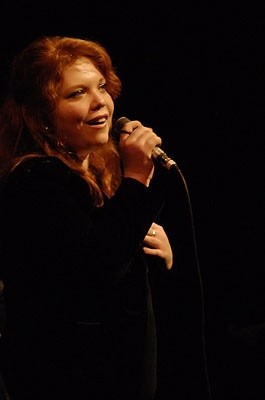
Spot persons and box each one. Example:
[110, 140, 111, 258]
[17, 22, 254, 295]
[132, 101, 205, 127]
[0, 35, 174, 400]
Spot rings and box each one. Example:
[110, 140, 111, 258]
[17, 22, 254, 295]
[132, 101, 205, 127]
[151, 229, 156, 237]
[120, 130, 133, 135]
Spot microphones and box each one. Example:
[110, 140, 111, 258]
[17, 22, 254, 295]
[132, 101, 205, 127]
[115, 117, 176, 171]
